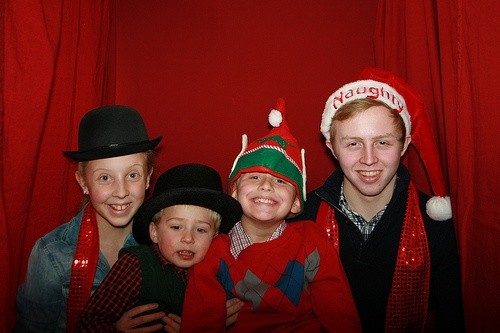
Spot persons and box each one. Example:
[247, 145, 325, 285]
[74, 163, 243, 333]
[15, 106, 182, 333]
[180, 109, 362, 333]
[226, 69, 466, 333]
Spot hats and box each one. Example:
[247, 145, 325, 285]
[132, 164, 243, 248]
[63, 105, 162, 162]
[227, 98, 306, 218]
[319, 66, 453, 221]
